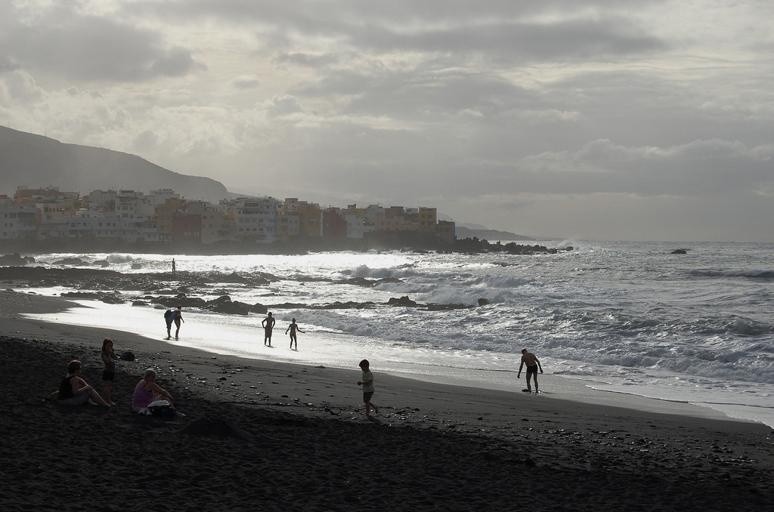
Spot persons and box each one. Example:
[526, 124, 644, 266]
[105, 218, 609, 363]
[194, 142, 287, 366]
[131, 369, 186, 417]
[172, 258, 175, 272]
[262, 312, 275, 347]
[57, 360, 109, 407]
[164, 310, 173, 339]
[99, 338, 119, 406]
[517, 349, 543, 394]
[285, 318, 305, 351]
[357, 359, 378, 415]
[173, 306, 184, 341]
